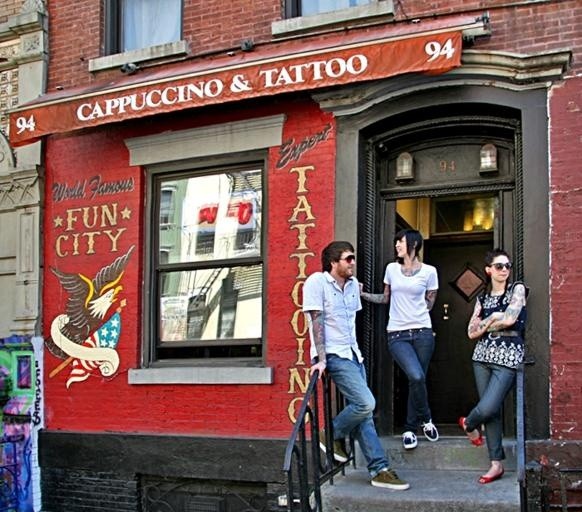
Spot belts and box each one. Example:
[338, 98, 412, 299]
[486, 329, 520, 338]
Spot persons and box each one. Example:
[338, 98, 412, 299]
[459, 248, 529, 483]
[303, 241, 410, 491]
[359, 229, 439, 450]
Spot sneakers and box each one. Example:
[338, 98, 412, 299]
[402, 431, 419, 451]
[370, 467, 411, 491]
[319, 429, 349, 462]
[420, 419, 441, 442]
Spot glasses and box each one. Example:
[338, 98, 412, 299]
[489, 261, 513, 271]
[338, 255, 356, 265]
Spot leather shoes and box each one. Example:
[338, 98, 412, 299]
[457, 415, 485, 446]
[477, 469, 505, 484]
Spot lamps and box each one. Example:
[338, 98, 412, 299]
[478, 143, 499, 176]
[394, 152, 416, 183]
